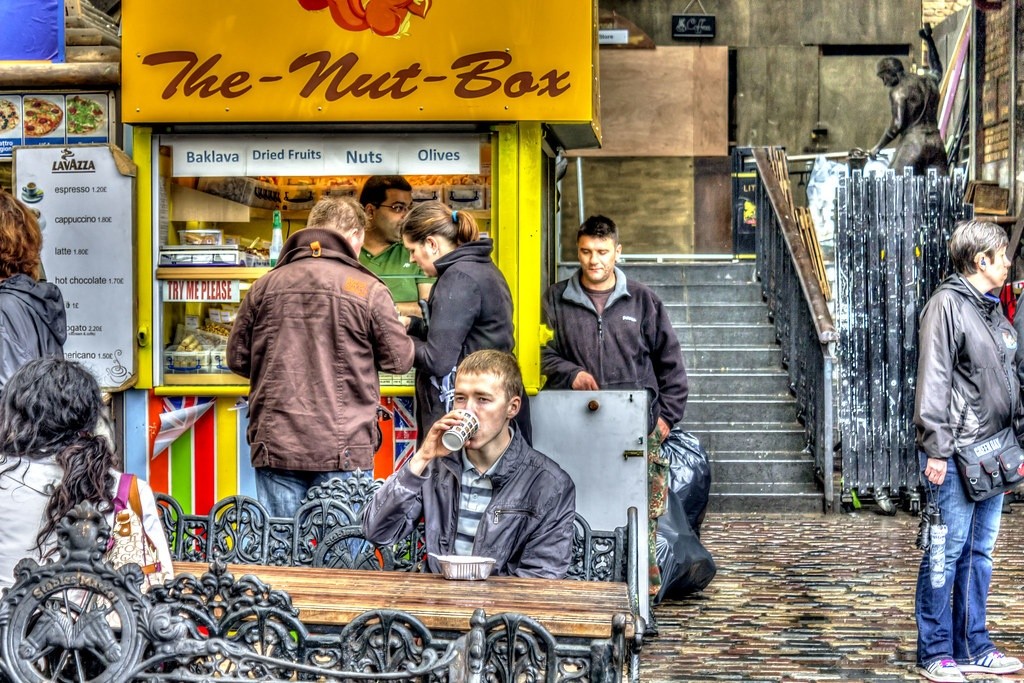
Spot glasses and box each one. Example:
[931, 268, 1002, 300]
[378, 204, 414, 213]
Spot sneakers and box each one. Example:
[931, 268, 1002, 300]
[915, 657, 964, 683]
[956, 650, 1023, 674]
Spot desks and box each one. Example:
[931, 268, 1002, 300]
[148, 562, 634, 683]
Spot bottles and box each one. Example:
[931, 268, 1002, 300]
[269, 210, 283, 268]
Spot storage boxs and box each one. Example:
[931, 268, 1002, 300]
[197, 175, 491, 210]
[164, 343, 227, 369]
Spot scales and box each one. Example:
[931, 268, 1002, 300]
[159, 244, 246, 267]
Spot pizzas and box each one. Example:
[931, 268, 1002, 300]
[0, 99, 19, 133]
[23, 97, 62, 136]
[66, 96, 105, 135]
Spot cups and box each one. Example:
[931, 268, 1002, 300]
[28, 207, 41, 221]
[443, 408, 480, 452]
[21, 182, 38, 197]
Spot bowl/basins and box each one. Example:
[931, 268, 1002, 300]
[437, 555, 497, 581]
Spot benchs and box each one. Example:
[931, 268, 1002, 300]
[0, 467, 645, 683]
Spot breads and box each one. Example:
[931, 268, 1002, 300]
[211, 175, 481, 188]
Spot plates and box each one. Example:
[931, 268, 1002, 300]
[36, 215, 47, 229]
[21, 189, 44, 202]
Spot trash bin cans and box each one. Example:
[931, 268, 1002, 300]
[731, 146, 787, 260]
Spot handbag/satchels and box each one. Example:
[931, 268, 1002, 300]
[953, 427, 1024, 502]
[97, 473, 165, 631]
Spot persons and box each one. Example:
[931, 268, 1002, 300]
[0, 361, 176, 683]
[913, 220, 1024, 683]
[0, 187, 67, 397]
[226, 175, 532, 569]
[868, 22, 947, 176]
[540, 215, 689, 637]
[361, 350, 576, 580]
